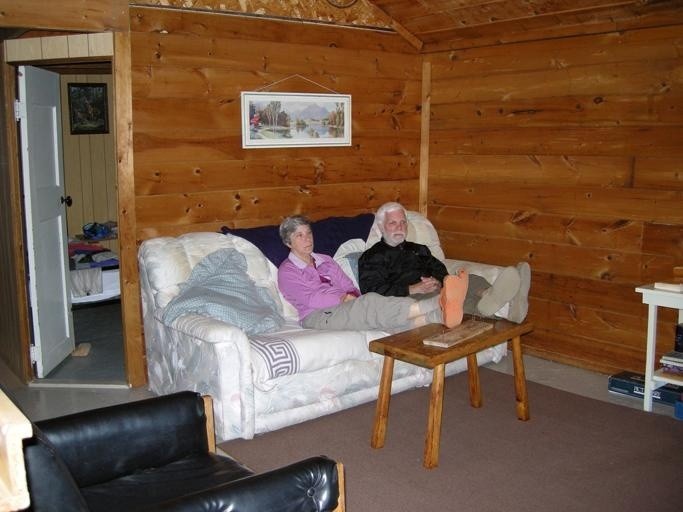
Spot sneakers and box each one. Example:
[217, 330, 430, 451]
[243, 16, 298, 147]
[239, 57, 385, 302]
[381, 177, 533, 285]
[476, 262, 531, 324]
[438, 267, 469, 329]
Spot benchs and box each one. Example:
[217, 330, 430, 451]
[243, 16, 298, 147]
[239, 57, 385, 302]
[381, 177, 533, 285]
[369, 313, 536, 468]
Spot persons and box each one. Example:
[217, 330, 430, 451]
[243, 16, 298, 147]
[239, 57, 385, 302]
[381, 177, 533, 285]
[355, 200, 531, 325]
[273, 213, 470, 335]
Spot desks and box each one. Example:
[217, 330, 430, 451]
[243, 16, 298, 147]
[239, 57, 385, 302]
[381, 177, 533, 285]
[635, 283, 683, 412]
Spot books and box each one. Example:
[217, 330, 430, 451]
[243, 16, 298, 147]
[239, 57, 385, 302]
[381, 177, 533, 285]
[652, 276, 682, 293]
[657, 349, 682, 377]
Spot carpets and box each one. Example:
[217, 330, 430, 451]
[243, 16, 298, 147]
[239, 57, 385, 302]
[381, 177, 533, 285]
[217, 363, 683, 512]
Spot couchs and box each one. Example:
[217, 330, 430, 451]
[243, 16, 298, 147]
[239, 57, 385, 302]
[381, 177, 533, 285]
[138, 209, 511, 442]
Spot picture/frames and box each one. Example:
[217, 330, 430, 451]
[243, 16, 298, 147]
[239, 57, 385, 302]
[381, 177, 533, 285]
[241, 91, 352, 150]
[67, 82, 109, 135]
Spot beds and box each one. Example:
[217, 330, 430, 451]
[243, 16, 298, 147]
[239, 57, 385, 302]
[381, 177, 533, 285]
[68, 241, 121, 303]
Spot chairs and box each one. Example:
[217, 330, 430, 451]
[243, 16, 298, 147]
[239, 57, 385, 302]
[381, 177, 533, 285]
[1, 388, 346, 512]
